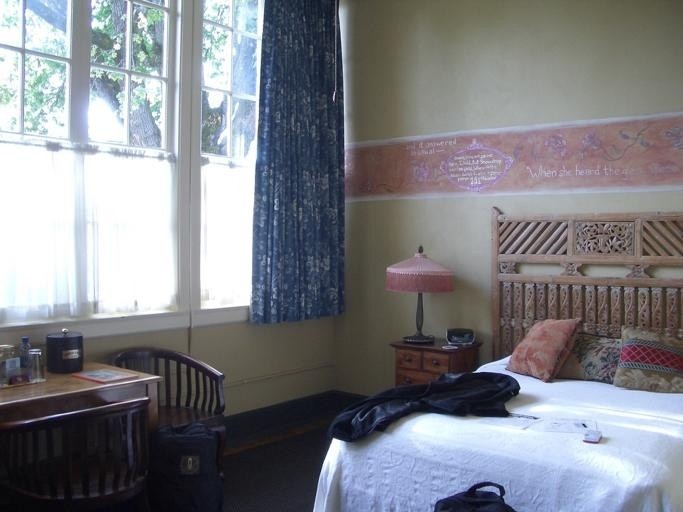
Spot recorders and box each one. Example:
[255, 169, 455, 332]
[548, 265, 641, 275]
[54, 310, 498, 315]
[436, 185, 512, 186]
[446, 328, 475, 345]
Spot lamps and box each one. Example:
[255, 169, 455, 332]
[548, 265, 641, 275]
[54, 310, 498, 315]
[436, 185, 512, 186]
[383, 244, 454, 346]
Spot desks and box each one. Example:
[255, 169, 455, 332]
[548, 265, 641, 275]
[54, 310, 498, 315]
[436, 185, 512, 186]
[1, 361, 163, 511]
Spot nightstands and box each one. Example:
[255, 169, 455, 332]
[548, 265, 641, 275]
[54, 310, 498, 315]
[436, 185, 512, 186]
[389, 338, 482, 386]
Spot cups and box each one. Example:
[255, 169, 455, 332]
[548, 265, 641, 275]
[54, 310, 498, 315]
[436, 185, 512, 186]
[26, 348, 46, 383]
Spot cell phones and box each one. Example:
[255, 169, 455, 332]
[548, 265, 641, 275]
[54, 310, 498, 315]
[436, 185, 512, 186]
[583, 429, 602, 442]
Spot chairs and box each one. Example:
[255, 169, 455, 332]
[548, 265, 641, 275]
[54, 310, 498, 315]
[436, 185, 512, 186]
[0, 395, 151, 510]
[110, 344, 230, 484]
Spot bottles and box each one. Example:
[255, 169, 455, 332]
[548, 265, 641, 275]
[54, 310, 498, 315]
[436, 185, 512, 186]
[18, 336, 32, 384]
[0, 344, 18, 388]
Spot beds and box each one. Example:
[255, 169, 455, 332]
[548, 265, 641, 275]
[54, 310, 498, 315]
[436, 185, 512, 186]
[312, 202, 682, 511]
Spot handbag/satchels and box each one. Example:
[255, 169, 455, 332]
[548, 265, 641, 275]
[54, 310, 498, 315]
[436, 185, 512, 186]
[433, 479, 517, 512]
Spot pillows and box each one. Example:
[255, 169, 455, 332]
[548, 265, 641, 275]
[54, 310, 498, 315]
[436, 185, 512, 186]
[611, 324, 683, 392]
[502, 316, 583, 383]
[555, 331, 622, 384]
